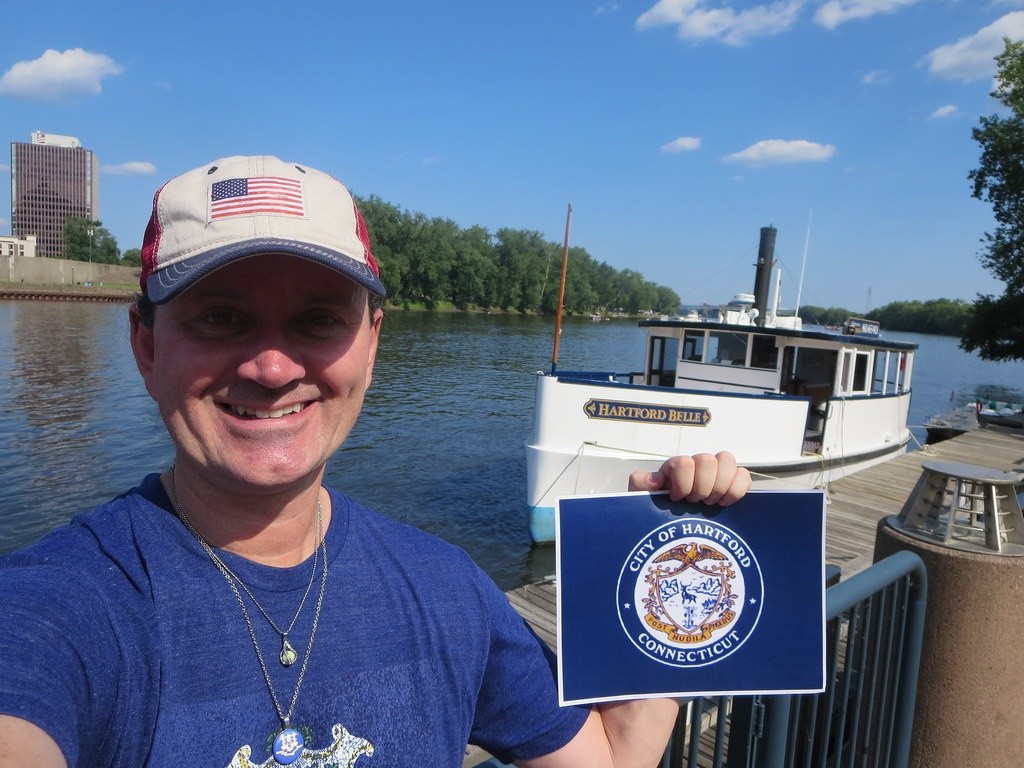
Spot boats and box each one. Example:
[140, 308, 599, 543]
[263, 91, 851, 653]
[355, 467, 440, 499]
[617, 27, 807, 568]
[717, 294, 803, 330]
[589, 313, 602, 320]
[522, 226, 922, 545]
[975, 407, 1024, 429]
[647, 310, 711, 322]
[842, 316, 880, 339]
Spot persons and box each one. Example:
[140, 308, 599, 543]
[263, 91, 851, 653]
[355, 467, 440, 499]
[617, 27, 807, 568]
[0, 155, 752, 768]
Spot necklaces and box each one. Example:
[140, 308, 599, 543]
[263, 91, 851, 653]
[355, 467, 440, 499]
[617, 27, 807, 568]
[170, 464, 328, 765]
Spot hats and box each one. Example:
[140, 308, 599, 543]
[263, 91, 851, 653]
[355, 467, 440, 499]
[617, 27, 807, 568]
[139, 155, 388, 307]
